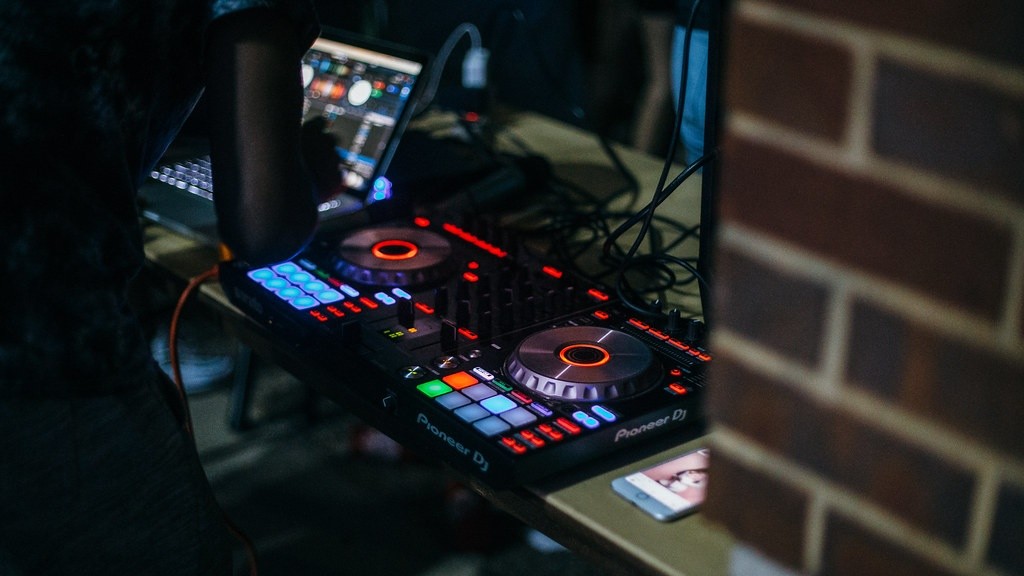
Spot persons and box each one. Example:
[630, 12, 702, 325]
[0, 3, 351, 576]
[657, 467, 709, 496]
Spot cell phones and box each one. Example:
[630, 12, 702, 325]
[609, 445, 708, 524]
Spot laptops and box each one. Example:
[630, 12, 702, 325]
[133, 24, 437, 251]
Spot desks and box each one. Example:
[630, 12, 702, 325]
[141, 104, 731, 576]
